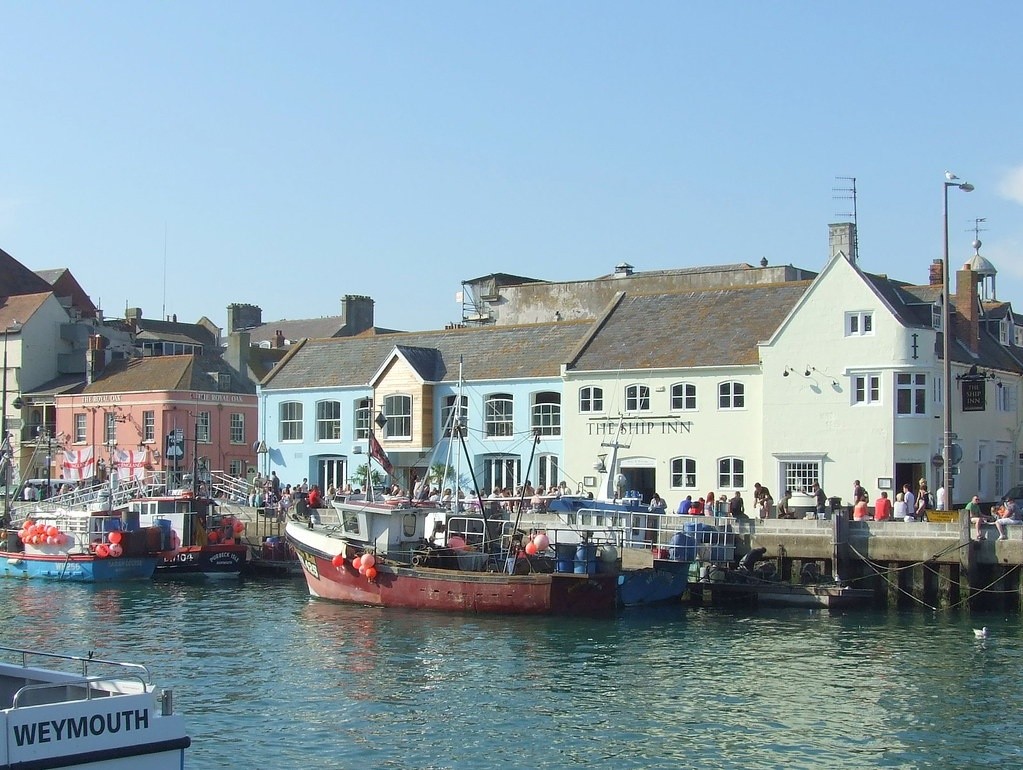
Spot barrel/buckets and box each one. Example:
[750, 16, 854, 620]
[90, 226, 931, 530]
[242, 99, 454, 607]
[102, 518, 120, 548]
[121, 511, 139, 532]
[265, 537, 279, 543]
[806, 512, 814, 520]
[817, 513, 825, 520]
[573, 543, 597, 574]
[153, 519, 171, 551]
[555, 543, 578, 573]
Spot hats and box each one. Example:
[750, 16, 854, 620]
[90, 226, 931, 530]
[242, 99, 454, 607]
[1005, 497, 1014, 502]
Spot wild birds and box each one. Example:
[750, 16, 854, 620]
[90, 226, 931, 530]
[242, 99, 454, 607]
[972, 626, 988, 637]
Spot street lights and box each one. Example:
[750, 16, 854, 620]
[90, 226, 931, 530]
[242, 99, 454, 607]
[944, 181, 974, 511]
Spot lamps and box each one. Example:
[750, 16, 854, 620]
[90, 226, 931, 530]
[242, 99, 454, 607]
[805, 365, 839, 388]
[784, 366, 818, 389]
[987, 377, 1003, 389]
[984, 368, 995, 380]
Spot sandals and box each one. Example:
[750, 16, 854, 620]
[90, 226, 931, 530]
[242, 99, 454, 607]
[977, 535, 986, 540]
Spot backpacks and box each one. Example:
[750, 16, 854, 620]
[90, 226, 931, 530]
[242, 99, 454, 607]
[691, 501, 700, 508]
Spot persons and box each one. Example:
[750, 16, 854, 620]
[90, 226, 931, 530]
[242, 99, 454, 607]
[802, 482, 828, 520]
[12, 474, 109, 503]
[853, 480, 871, 522]
[224, 523, 234, 545]
[777, 490, 797, 520]
[676, 492, 750, 520]
[384, 475, 595, 513]
[754, 482, 774, 519]
[199, 479, 210, 496]
[874, 478, 945, 523]
[647, 491, 668, 549]
[965, 495, 1022, 540]
[220, 471, 366, 520]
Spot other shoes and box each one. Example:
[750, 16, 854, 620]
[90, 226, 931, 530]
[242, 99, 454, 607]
[998, 534, 1006, 539]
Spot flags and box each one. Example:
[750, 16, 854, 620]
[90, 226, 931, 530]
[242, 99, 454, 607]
[64, 444, 94, 480]
[112, 449, 147, 482]
[370, 432, 393, 475]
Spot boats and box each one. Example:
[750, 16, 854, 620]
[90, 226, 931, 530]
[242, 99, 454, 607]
[0, 522, 163, 585]
[283, 515, 622, 618]
[0, 641, 193, 770]
[686, 556, 875, 611]
[0, 385, 694, 609]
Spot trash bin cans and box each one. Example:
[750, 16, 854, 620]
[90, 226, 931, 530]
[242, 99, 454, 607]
[7, 531, 19, 552]
[574, 543, 598, 574]
[153, 519, 171, 551]
[146, 527, 161, 553]
[555, 542, 577, 573]
[125, 532, 147, 555]
